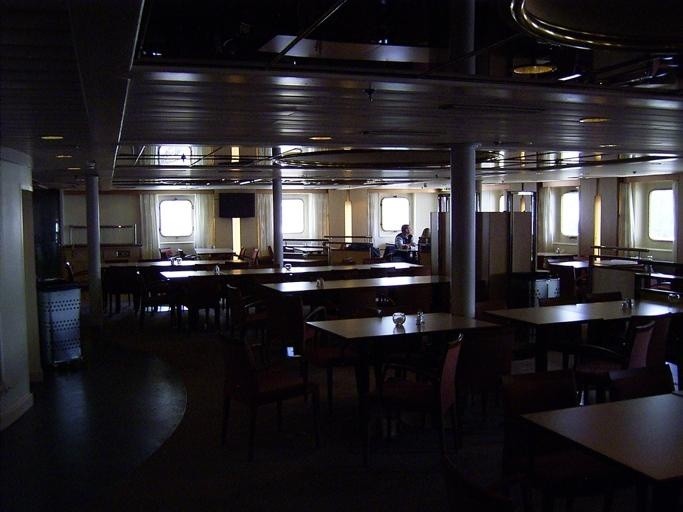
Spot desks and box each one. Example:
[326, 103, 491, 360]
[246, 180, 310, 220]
[524, 388, 683, 512]
[393, 245, 420, 264]
[193, 247, 238, 260]
[304, 311, 499, 428]
[537, 248, 578, 268]
[98, 258, 249, 269]
[633, 268, 683, 301]
[483, 299, 683, 373]
[295, 247, 324, 256]
[265, 273, 453, 298]
[158, 262, 424, 281]
[551, 258, 646, 298]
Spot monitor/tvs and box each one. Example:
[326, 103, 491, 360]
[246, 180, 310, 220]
[219, 192, 255, 218]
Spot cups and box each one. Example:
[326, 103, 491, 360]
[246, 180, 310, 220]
[393, 313, 406, 325]
[285, 264, 291, 270]
[177, 258, 182, 264]
[393, 325, 405, 334]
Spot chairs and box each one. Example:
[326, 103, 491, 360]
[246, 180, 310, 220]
[244, 248, 259, 268]
[416, 242, 431, 266]
[472, 301, 535, 359]
[107, 267, 137, 314]
[60, 262, 90, 288]
[588, 323, 656, 407]
[611, 364, 675, 401]
[221, 283, 271, 343]
[329, 269, 360, 283]
[224, 332, 326, 468]
[385, 242, 401, 262]
[233, 246, 248, 260]
[502, 367, 618, 512]
[159, 246, 177, 260]
[183, 275, 220, 328]
[624, 314, 671, 363]
[557, 263, 588, 302]
[134, 269, 183, 325]
[264, 294, 328, 344]
[177, 247, 199, 261]
[358, 329, 463, 466]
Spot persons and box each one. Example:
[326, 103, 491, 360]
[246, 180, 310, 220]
[418, 228, 431, 248]
[395, 224, 416, 249]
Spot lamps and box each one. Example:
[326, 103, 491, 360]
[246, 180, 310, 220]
[511, 50, 556, 77]
